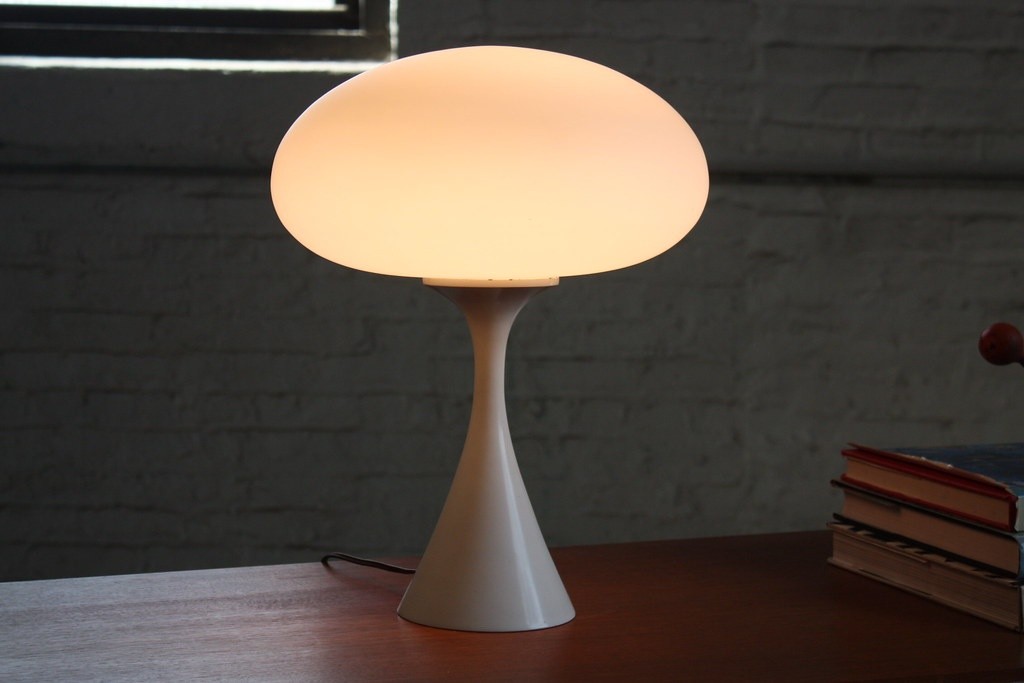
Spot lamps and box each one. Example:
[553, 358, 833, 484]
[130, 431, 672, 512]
[268, 44, 710, 632]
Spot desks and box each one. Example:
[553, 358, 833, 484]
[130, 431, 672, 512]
[1, 529, 1024, 683]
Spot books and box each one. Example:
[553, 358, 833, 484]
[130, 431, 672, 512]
[825, 440, 1024, 628]
